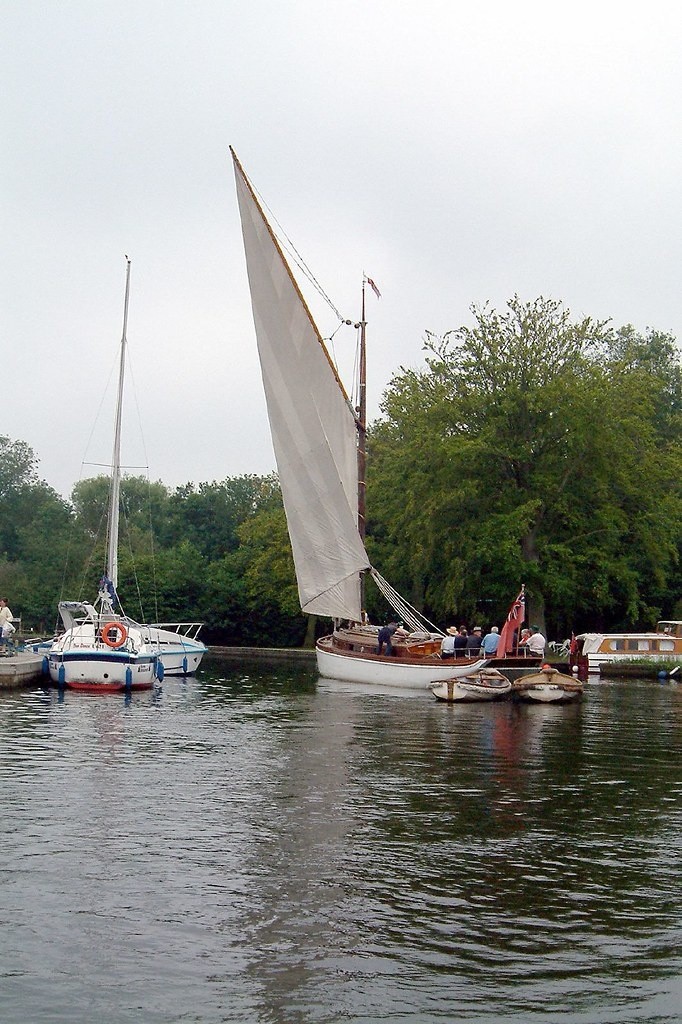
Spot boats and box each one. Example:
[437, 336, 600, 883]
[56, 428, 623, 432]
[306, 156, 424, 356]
[514, 665, 584, 704]
[570, 620, 682, 675]
[427, 669, 514, 703]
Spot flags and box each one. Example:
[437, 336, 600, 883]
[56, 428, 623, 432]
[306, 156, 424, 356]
[495, 590, 525, 660]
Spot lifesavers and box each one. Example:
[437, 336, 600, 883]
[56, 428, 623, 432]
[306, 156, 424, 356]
[102, 623, 126, 647]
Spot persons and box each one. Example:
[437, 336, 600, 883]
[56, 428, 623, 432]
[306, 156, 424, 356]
[441, 625, 545, 660]
[0, 597, 13, 657]
[377, 623, 406, 656]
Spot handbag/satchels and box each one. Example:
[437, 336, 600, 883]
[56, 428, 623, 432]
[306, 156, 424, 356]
[2, 621, 16, 638]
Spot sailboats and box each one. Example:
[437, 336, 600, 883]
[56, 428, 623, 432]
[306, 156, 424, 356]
[228, 143, 543, 692]
[23, 253, 208, 693]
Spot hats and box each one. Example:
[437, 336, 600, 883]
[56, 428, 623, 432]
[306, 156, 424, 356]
[446, 627, 458, 635]
[471, 627, 484, 634]
[529, 625, 540, 632]
